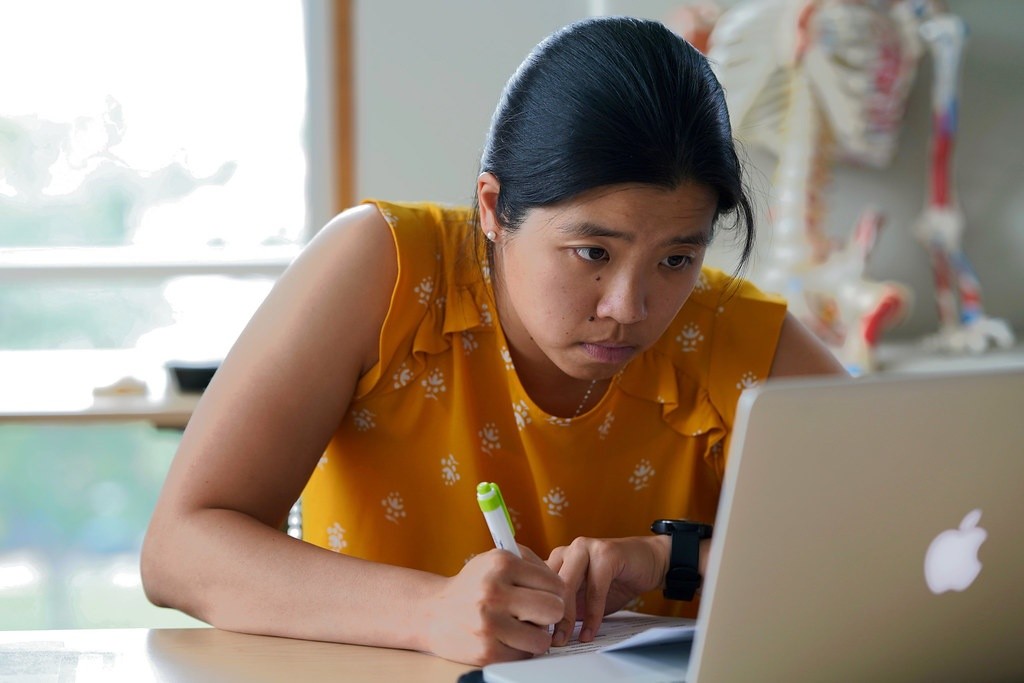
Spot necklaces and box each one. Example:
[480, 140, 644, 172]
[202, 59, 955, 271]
[573, 381, 597, 419]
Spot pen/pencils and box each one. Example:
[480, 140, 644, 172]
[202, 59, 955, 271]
[473, 480, 553, 656]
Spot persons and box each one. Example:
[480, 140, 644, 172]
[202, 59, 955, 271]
[140, 15, 855, 667]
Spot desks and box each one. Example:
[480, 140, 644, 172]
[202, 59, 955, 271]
[0, 590, 483, 683]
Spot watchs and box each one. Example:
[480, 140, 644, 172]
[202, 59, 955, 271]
[650, 519, 713, 602]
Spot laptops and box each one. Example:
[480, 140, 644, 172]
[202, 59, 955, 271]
[483, 356, 1024, 683]
